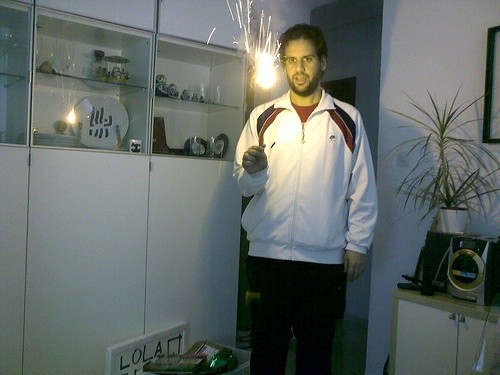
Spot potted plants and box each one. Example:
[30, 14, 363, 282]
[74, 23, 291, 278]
[376, 82, 500, 234]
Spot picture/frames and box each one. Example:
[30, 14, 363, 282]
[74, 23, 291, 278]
[481, 26, 500, 143]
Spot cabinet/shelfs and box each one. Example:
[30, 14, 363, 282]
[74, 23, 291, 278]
[0, 1, 250, 375]
[388, 289, 500, 375]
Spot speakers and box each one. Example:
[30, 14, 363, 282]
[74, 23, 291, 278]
[447, 236, 500, 306]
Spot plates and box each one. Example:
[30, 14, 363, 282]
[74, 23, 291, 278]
[71, 95, 129, 149]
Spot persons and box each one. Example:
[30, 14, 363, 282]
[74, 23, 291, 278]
[232, 24, 379, 375]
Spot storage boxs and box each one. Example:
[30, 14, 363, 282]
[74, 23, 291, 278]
[139, 342, 252, 375]
